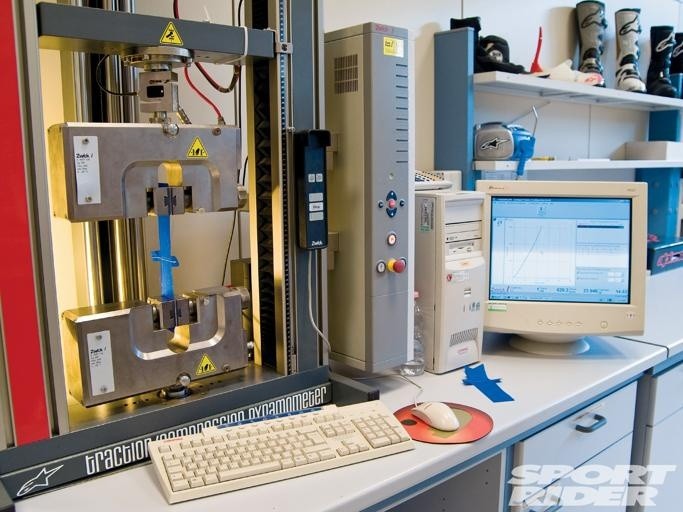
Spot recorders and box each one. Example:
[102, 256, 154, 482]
[473, 100, 551, 162]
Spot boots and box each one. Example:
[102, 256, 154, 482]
[449, 16, 525, 74]
[575, 0, 607, 88]
[614, 7, 646, 93]
[647, 26, 683, 99]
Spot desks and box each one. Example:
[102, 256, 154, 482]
[0, 270, 683, 512]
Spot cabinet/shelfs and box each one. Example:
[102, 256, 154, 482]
[435, 1, 683, 270]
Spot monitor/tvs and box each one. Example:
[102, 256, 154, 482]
[475, 179, 648, 358]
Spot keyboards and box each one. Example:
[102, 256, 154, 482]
[147, 398, 416, 504]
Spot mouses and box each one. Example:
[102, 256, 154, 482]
[411, 401, 460, 431]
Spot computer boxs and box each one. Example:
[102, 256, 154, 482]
[413, 188, 488, 375]
